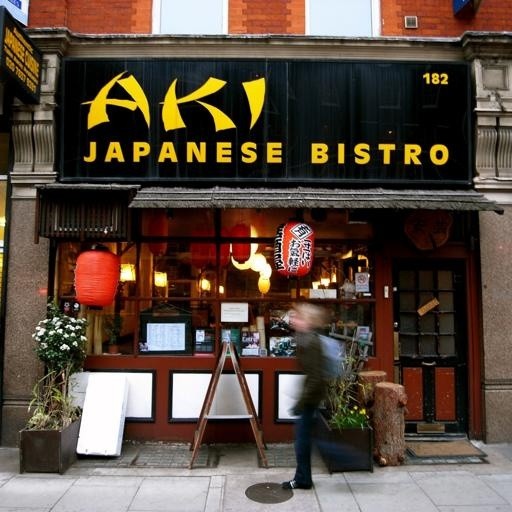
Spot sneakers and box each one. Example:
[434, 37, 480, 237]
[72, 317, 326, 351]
[282, 480, 312, 490]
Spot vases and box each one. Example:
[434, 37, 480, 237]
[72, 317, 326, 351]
[316, 406, 374, 475]
[18, 409, 81, 475]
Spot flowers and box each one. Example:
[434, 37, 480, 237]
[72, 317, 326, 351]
[28, 297, 89, 428]
[327, 328, 373, 432]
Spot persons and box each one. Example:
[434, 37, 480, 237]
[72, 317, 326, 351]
[282, 302, 341, 488]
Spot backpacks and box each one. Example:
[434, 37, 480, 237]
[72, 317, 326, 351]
[317, 333, 342, 379]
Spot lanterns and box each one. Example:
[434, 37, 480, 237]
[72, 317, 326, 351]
[74, 245, 121, 310]
[274, 216, 315, 281]
[191, 223, 250, 269]
[147, 212, 170, 257]
